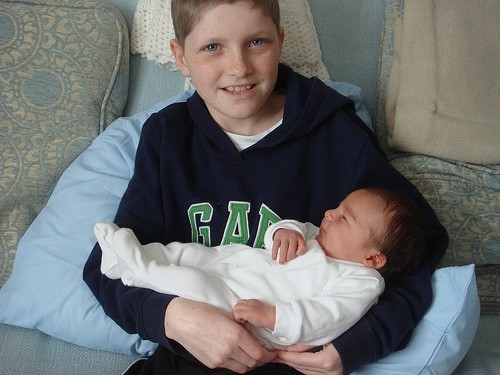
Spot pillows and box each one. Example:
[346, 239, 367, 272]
[0, 82, 480, 375]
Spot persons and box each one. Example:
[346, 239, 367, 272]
[93, 188, 430, 351]
[82, 0, 449, 375]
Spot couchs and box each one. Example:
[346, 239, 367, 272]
[0, 0, 500, 375]
